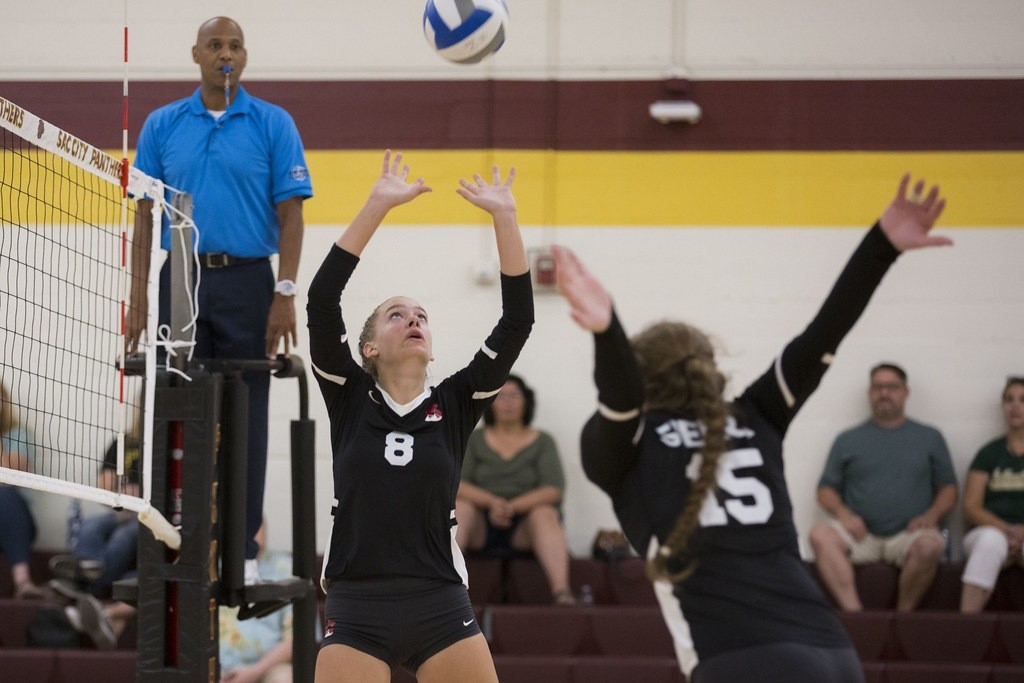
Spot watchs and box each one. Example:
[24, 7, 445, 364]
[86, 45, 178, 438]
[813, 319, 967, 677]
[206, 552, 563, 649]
[274, 279, 298, 297]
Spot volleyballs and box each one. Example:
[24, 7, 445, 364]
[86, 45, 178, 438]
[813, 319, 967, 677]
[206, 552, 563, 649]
[421, 0, 511, 65]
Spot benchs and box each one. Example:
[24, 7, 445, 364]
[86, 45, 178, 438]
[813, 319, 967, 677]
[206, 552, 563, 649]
[0, 554, 1024, 682]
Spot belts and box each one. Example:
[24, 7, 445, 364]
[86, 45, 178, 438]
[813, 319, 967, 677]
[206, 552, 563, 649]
[193, 253, 253, 269]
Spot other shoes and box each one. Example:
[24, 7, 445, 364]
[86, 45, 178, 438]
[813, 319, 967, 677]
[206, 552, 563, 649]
[9, 543, 134, 652]
[551, 589, 577, 609]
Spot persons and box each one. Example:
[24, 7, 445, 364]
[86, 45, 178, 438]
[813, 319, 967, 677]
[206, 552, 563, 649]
[809, 363, 960, 612]
[455, 374, 575, 605]
[961, 376, 1024, 611]
[304, 149, 535, 683]
[552, 172, 956, 683]
[0, 383, 43, 599]
[123, 15, 313, 582]
[50, 423, 266, 651]
